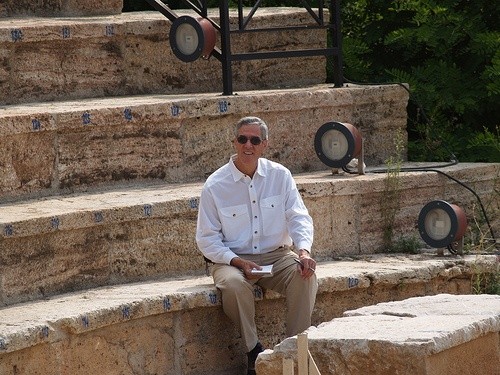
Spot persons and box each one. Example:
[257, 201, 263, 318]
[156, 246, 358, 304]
[196, 116, 320, 374]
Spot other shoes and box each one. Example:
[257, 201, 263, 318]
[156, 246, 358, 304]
[247, 343, 263, 375]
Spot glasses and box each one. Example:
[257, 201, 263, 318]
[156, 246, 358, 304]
[235, 134, 266, 146]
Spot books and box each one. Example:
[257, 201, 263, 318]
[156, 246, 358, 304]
[250, 265, 274, 278]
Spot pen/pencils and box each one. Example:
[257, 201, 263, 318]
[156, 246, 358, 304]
[294, 258, 316, 273]
